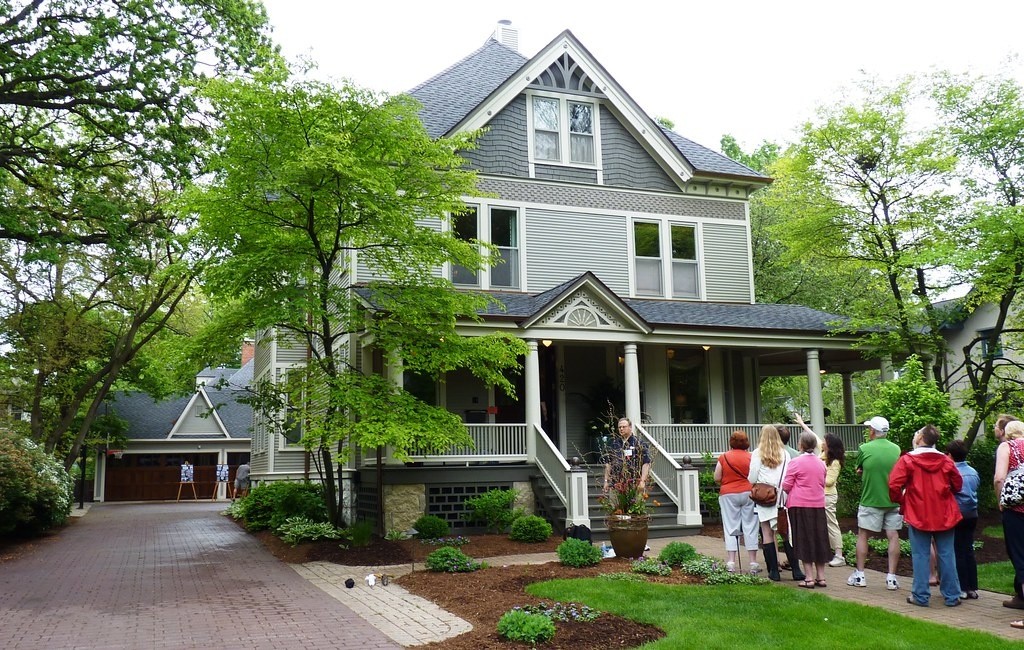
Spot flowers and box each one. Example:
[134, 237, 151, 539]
[570, 400, 662, 525]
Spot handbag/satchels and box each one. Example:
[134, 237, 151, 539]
[999, 441, 1024, 509]
[776, 507, 788, 540]
[749, 483, 776, 507]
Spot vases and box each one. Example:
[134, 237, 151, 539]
[609, 524, 649, 559]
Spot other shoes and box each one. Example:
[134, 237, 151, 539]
[929, 576, 937, 586]
[960, 590, 968, 599]
[1003, 598, 1024, 610]
[907, 597, 929, 606]
[1010, 620, 1024, 629]
[967, 590, 978, 599]
[726, 557, 827, 591]
[644, 545, 651, 550]
[944, 599, 962, 607]
[937, 576, 941, 585]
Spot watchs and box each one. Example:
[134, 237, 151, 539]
[605, 480, 607, 481]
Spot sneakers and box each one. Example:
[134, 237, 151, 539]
[828, 553, 846, 567]
[885, 578, 900, 590]
[846, 569, 867, 586]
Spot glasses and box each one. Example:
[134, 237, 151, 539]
[994, 424, 1000, 429]
[617, 426, 628, 429]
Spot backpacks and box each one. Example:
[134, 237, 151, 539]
[562, 524, 593, 546]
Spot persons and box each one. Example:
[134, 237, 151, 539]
[232, 462, 250, 501]
[540, 375, 548, 422]
[945, 439, 980, 599]
[783, 432, 827, 587]
[747, 423, 801, 581]
[793, 413, 844, 568]
[889, 423, 963, 606]
[994, 420, 1024, 609]
[846, 416, 902, 590]
[713, 430, 764, 573]
[605, 418, 651, 550]
[994, 413, 1019, 441]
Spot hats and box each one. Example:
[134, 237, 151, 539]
[864, 416, 891, 432]
